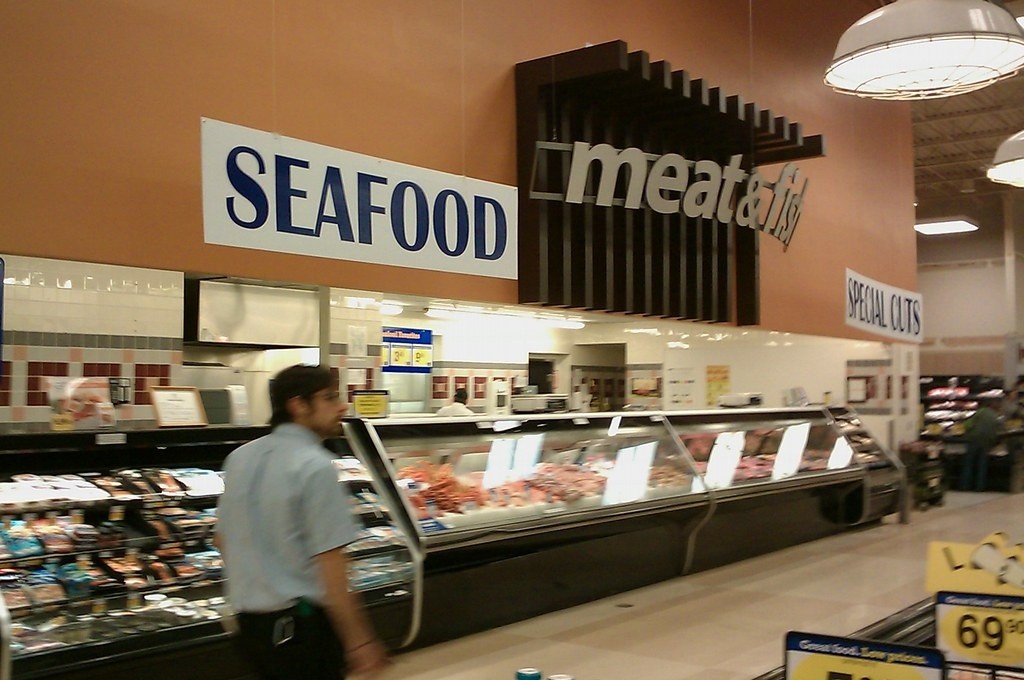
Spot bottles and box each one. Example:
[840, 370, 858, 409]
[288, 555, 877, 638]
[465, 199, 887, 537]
[516, 667, 575, 680]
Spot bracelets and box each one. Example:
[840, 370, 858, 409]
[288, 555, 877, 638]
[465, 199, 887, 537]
[343, 636, 376, 655]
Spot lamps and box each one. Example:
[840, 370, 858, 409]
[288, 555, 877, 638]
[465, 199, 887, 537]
[988, 127, 1024, 187]
[824, 0, 1024, 101]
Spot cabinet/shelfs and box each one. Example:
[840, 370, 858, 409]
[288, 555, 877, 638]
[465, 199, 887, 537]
[1, 419, 426, 680]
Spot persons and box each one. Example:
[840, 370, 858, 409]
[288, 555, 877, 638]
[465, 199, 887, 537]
[214, 364, 386, 680]
[429, 388, 476, 476]
[962, 397, 1004, 493]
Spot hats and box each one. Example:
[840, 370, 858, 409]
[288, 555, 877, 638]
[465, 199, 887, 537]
[268, 366, 332, 426]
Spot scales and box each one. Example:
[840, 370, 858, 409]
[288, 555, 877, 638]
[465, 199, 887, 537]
[511, 394, 569, 411]
[719, 392, 762, 407]
